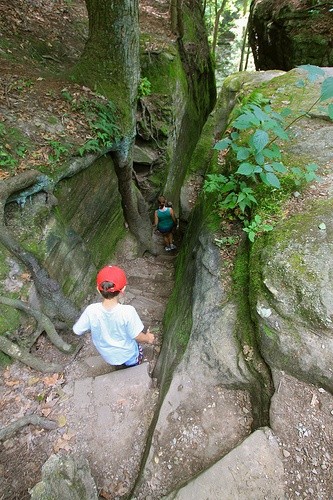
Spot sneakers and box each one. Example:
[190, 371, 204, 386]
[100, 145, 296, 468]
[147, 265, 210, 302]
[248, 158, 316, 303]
[170, 244, 176, 249]
[164, 246, 171, 251]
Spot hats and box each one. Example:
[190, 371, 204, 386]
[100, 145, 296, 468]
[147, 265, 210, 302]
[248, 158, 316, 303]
[96, 265, 128, 294]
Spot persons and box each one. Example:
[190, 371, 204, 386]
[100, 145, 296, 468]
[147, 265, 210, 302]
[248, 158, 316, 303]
[168, 202, 177, 228]
[154, 196, 176, 251]
[72, 263, 154, 370]
